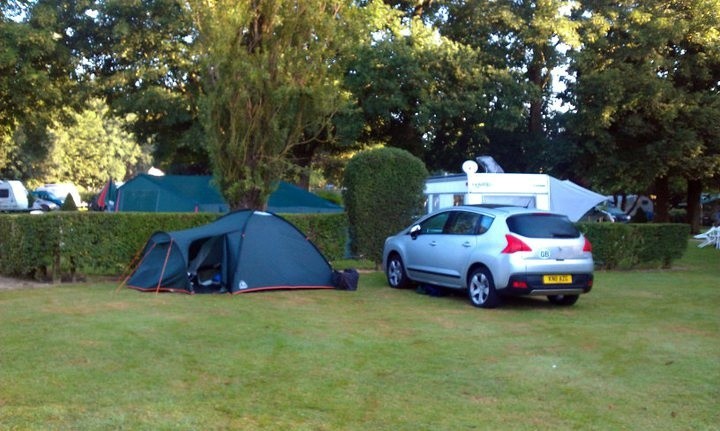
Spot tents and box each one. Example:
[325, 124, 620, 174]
[114, 170, 342, 216]
[116, 207, 331, 298]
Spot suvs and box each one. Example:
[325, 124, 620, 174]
[382, 197, 596, 309]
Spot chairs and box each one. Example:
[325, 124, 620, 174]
[691, 225, 720, 252]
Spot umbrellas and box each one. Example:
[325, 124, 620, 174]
[92, 176, 118, 211]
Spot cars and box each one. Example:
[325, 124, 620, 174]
[0, 180, 81, 212]
